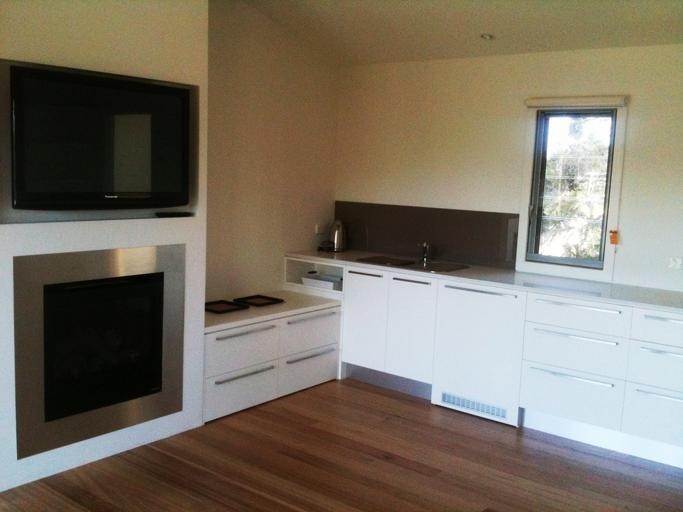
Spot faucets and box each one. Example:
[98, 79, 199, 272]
[417, 241, 433, 263]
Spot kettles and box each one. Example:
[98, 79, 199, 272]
[329, 219, 349, 254]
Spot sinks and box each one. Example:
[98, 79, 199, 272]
[356, 256, 419, 266]
[405, 262, 472, 273]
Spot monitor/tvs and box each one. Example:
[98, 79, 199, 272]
[10, 65, 190, 211]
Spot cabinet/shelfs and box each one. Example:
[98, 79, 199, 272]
[434, 274, 526, 429]
[285, 254, 343, 299]
[524, 288, 633, 447]
[202, 302, 341, 424]
[630, 304, 683, 466]
[344, 263, 438, 388]
